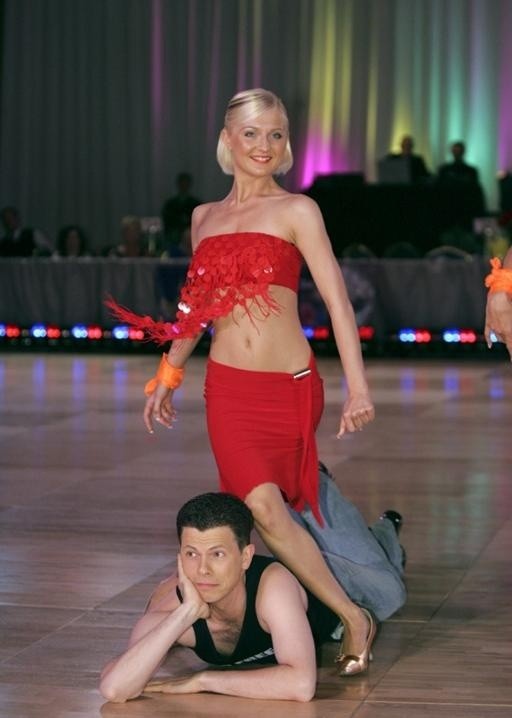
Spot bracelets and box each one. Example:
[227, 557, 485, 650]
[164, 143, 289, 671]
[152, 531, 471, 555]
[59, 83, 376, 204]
[144, 353, 185, 396]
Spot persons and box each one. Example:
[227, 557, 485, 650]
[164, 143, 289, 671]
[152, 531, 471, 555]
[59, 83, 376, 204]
[100, 90, 378, 676]
[484, 245, 512, 363]
[98, 460, 407, 703]
[3, 172, 200, 260]
[302, 137, 511, 261]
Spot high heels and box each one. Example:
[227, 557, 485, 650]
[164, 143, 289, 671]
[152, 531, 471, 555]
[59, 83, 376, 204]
[334, 608, 377, 675]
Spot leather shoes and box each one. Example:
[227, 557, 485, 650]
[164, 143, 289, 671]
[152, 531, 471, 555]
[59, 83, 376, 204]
[381, 510, 406, 570]
